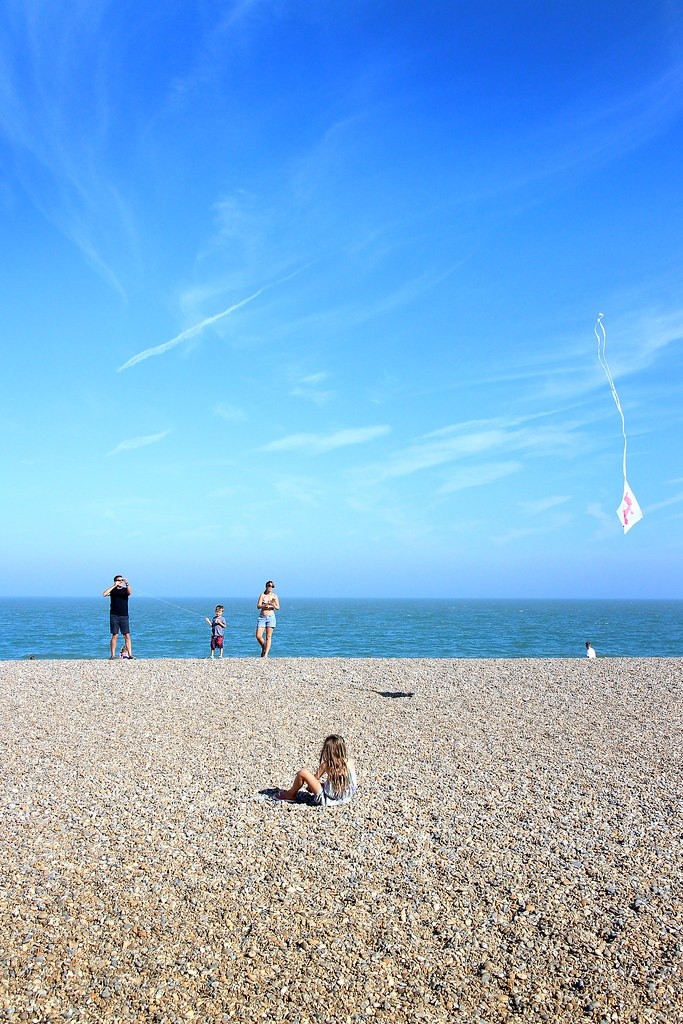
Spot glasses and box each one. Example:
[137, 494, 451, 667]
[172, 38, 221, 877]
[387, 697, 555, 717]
[116, 579, 124, 581]
[267, 585, 275, 588]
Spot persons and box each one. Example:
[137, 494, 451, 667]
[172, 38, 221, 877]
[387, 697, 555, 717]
[206, 605, 226, 658]
[103, 575, 135, 660]
[256, 581, 279, 656]
[273, 734, 358, 806]
[586, 642, 597, 658]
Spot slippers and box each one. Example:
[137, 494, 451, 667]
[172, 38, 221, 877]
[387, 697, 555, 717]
[273, 789, 296, 802]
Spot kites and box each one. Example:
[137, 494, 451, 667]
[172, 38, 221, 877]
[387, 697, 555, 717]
[592, 314, 644, 533]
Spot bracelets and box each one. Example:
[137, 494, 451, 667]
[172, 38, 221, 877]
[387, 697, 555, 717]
[125, 582, 129, 586]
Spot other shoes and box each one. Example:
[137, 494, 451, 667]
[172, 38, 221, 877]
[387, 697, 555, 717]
[219, 656, 222, 659]
[261, 650, 266, 657]
[109, 657, 114, 660]
[210, 656, 214, 658]
[129, 656, 136, 660]
